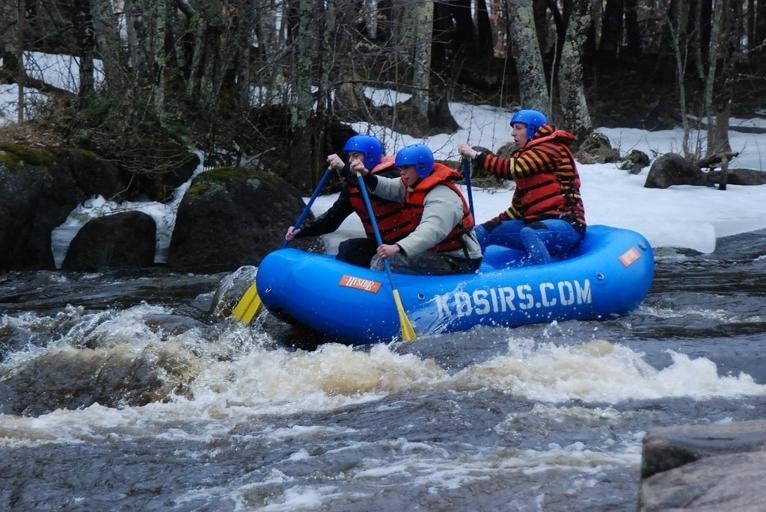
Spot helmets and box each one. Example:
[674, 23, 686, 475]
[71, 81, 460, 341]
[509, 110, 547, 141]
[394, 142, 435, 180]
[343, 134, 385, 170]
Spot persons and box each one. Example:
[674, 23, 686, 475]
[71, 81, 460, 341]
[350, 141, 484, 278]
[282, 134, 415, 269]
[458, 108, 588, 267]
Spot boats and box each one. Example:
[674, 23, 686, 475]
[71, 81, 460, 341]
[256, 223, 654, 347]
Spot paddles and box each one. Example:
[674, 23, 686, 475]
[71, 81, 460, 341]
[230, 160, 331, 324]
[353, 164, 417, 341]
